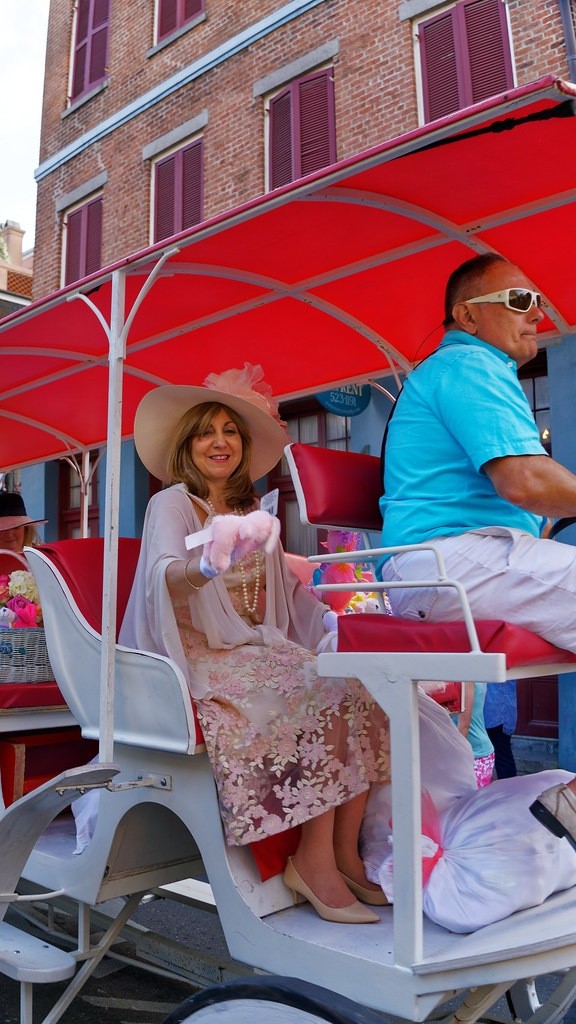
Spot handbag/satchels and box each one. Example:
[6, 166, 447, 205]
[387, 769, 576, 934]
[359, 686, 479, 885]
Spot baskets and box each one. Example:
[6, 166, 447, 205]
[0, 550, 55, 682]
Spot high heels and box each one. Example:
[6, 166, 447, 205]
[284, 856, 393, 923]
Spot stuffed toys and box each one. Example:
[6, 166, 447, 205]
[185, 510, 280, 578]
[0, 595, 38, 628]
[311, 531, 391, 650]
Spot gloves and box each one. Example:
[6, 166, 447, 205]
[322, 611, 338, 632]
[199, 515, 245, 578]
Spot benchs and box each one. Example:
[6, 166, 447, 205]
[283, 439, 576, 975]
[22, 537, 375, 919]
[0, 681, 99, 809]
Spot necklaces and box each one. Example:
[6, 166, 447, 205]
[207, 497, 260, 613]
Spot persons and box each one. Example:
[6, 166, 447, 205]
[450, 681, 517, 788]
[0, 492, 48, 558]
[376, 254, 576, 850]
[118, 366, 390, 924]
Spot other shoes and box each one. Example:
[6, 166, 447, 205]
[529, 783, 576, 849]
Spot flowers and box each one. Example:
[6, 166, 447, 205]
[0, 569, 43, 629]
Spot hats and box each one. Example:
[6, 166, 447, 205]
[134, 362, 288, 484]
[0, 493, 48, 531]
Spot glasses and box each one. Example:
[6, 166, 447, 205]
[466, 287, 541, 312]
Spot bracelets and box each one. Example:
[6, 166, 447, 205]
[184, 558, 206, 590]
